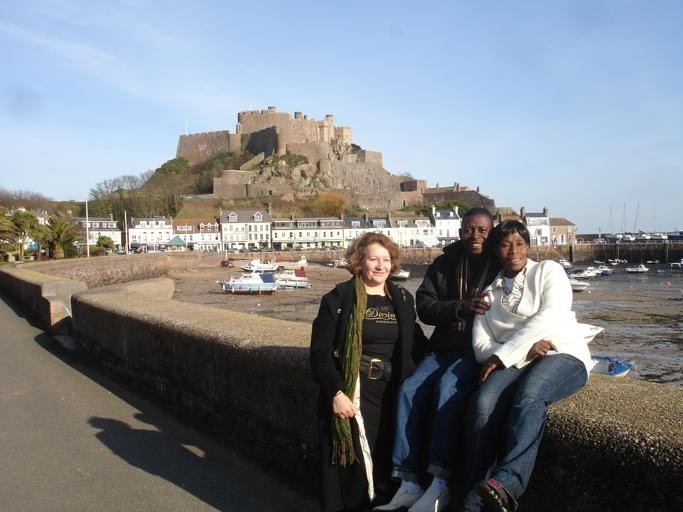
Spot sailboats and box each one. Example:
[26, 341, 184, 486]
[604, 196, 669, 242]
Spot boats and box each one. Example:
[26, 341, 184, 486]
[579, 323, 635, 378]
[218, 256, 312, 296]
[389, 268, 409, 280]
[559, 256, 683, 294]
[332, 257, 348, 267]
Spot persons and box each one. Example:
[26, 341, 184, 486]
[371, 207, 504, 512]
[463, 221, 594, 512]
[307, 232, 432, 512]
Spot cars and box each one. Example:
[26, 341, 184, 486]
[229, 245, 340, 254]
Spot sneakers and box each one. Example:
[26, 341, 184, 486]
[408, 483, 452, 512]
[474, 482, 514, 512]
[372, 484, 424, 510]
